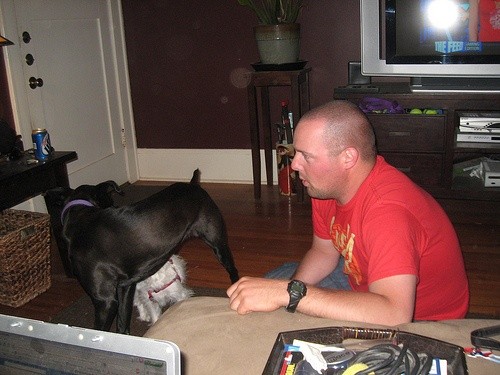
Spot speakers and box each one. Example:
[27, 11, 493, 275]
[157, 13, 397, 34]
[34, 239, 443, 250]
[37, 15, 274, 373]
[348, 62, 371, 86]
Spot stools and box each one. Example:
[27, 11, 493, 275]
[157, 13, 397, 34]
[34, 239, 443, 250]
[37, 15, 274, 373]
[245, 67, 311, 200]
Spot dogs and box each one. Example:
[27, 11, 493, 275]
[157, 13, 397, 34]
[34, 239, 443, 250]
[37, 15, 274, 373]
[46, 170, 241, 332]
[128, 255, 194, 322]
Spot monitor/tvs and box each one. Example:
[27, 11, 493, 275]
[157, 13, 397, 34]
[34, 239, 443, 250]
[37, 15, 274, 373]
[360, 0, 500, 93]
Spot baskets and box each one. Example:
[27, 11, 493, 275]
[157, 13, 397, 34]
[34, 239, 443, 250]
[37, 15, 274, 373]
[0, 209, 52, 308]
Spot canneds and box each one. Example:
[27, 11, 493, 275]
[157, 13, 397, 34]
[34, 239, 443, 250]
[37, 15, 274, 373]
[32, 128, 52, 161]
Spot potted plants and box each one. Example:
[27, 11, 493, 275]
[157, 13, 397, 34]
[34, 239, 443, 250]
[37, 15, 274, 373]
[240, 0, 305, 65]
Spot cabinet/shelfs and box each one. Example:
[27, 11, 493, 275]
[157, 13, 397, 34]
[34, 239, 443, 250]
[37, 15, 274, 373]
[332, 84, 500, 202]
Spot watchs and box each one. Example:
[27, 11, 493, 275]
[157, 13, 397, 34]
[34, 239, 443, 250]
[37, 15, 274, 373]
[286, 280, 307, 313]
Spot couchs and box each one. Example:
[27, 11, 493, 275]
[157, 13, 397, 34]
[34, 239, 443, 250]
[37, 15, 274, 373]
[141, 296, 500, 375]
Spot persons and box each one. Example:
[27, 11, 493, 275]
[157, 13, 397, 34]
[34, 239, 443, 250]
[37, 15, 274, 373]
[469, 0, 500, 43]
[227, 100, 470, 326]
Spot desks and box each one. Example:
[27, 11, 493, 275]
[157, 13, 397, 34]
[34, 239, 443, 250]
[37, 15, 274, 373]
[0, 151, 79, 280]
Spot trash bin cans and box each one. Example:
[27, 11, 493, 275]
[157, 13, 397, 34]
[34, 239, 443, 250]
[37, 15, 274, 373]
[0, 208, 53, 309]
